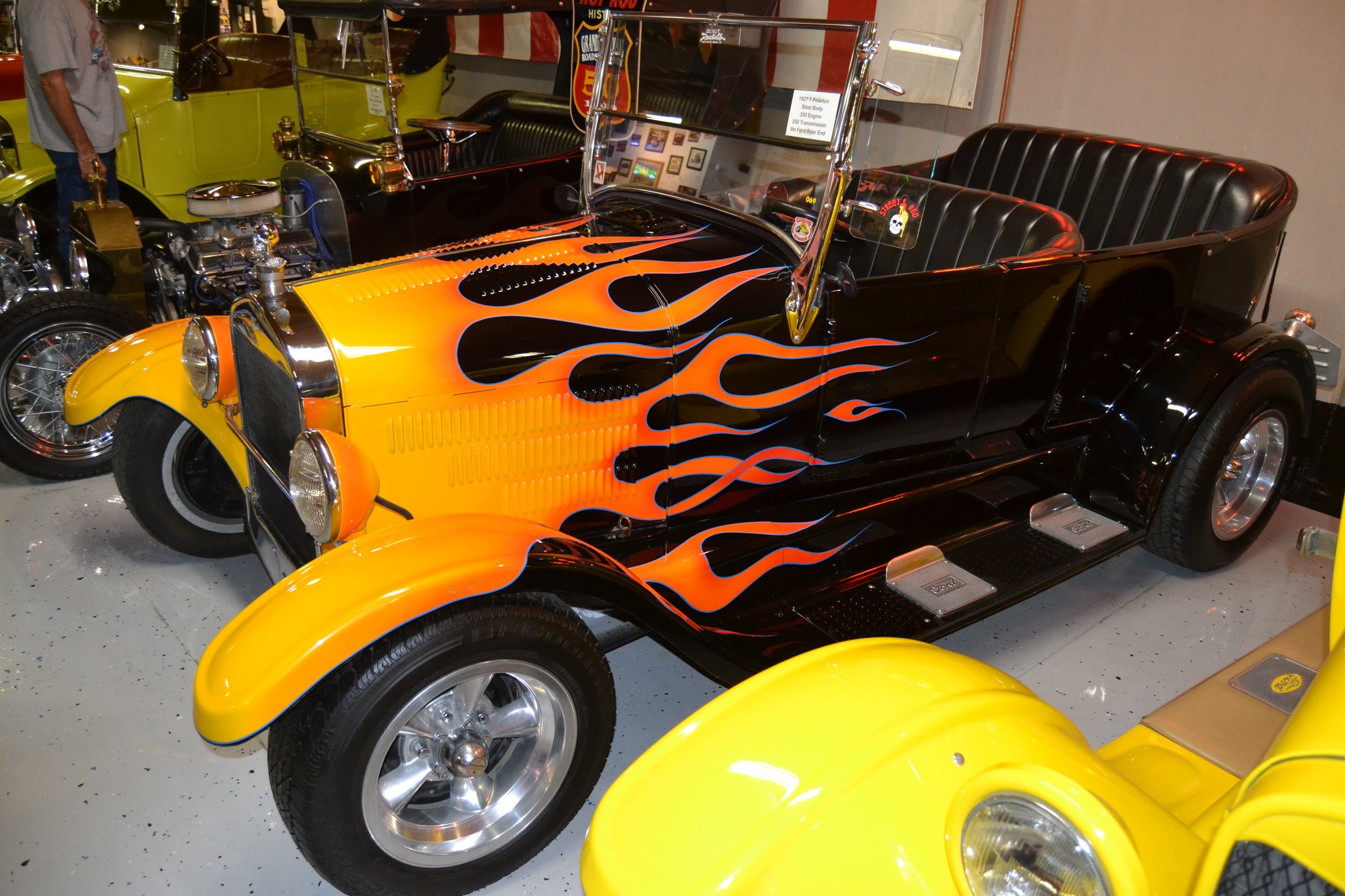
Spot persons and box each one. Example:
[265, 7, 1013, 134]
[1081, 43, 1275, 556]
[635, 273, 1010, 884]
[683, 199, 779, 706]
[17, 0, 130, 296]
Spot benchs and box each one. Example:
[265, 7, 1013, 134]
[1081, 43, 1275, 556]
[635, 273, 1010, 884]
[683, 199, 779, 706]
[768, 168, 1084, 281]
[192, 33, 308, 92]
[480, 90, 586, 165]
[944, 122, 1288, 251]
[337, 26, 420, 74]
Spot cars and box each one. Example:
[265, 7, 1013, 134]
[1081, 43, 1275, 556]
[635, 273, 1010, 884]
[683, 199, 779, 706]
[570, 485, 1345, 896]
[1, 1, 759, 478]
[54, 8, 1344, 896]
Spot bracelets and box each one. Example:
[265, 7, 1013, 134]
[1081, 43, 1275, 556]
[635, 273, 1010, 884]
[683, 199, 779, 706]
[79, 153, 97, 161]
